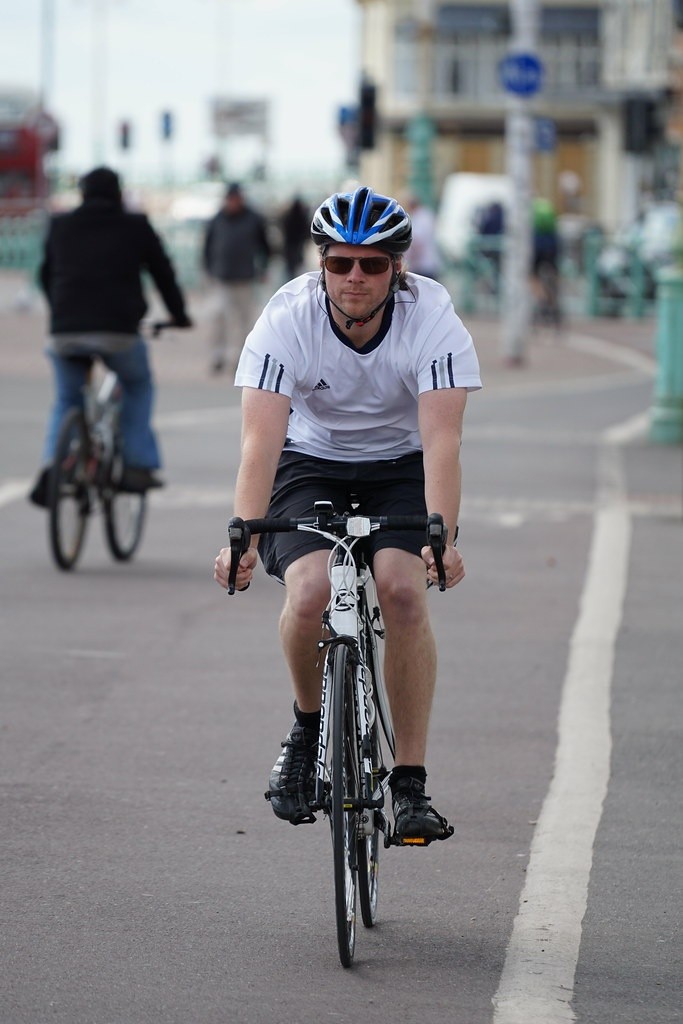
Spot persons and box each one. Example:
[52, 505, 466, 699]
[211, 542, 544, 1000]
[277, 200, 311, 281]
[201, 181, 271, 380]
[213, 186, 484, 840]
[394, 193, 440, 284]
[533, 201, 561, 326]
[29, 167, 190, 508]
[475, 202, 501, 265]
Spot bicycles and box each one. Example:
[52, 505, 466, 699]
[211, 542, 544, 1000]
[44, 319, 187, 571]
[228, 515, 451, 968]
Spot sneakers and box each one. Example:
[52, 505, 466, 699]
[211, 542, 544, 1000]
[268, 724, 318, 820]
[393, 778, 444, 836]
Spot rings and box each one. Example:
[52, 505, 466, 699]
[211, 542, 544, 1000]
[449, 575, 454, 581]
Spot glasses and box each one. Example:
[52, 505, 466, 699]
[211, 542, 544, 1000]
[320, 244, 395, 275]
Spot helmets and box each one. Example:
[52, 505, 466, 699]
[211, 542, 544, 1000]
[310, 187, 413, 254]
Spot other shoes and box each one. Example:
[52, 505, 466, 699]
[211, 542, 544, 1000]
[119, 467, 161, 491]
[31, 468, 49, 507]
